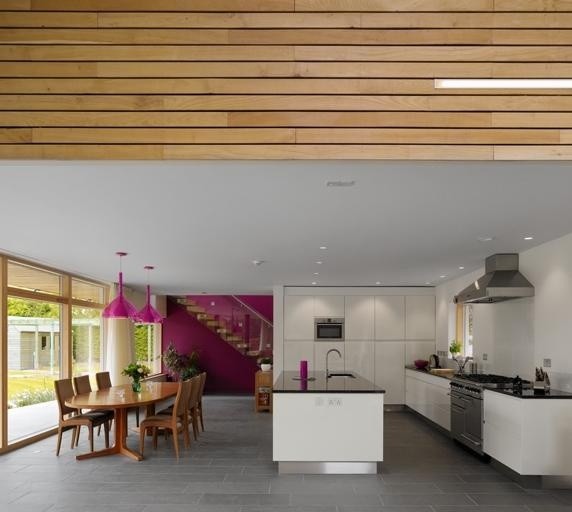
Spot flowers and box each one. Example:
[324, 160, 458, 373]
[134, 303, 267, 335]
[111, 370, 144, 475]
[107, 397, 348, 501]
[120, 363, 151, 382]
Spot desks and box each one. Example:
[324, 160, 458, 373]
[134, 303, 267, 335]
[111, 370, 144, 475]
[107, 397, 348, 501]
[255, 370, 272, 413]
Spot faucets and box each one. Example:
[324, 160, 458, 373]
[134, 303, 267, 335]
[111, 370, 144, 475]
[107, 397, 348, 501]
[326, 349, 341, 378]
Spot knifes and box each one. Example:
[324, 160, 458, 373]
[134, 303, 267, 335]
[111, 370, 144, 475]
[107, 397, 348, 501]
[535, 367, 544, 380]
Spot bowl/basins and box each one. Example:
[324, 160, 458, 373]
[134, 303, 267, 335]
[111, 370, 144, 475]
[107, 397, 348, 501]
[415, 360, 428, 368]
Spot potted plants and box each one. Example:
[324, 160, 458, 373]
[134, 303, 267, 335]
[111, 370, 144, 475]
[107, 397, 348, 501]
[261, 359, 271, 371]
[450, 342, 463, 360]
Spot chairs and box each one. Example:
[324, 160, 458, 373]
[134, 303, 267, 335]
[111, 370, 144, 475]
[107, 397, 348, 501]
[54, 372, 140, 456]
[139, 372, 206, 459]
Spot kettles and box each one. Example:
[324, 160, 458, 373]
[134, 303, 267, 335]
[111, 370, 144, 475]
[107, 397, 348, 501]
[429, 354, 439, 369]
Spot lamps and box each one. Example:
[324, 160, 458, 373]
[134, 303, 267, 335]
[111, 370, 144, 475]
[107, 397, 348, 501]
[134, 266, 164, 323]
[101, 252, 138, 319]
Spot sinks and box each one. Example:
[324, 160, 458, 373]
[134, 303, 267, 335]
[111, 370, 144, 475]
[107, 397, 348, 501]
[330, 373, 354, 379]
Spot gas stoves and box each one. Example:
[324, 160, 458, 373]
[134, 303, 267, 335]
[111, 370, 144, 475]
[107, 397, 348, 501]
[451, 373, 532, 399]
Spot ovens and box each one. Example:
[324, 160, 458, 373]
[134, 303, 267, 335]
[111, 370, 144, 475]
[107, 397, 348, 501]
[451, 390, 484, 456]
[314, 317, 345, 342]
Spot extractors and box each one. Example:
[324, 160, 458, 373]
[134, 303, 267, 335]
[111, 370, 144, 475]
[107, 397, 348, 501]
[453, 254, 535, 303]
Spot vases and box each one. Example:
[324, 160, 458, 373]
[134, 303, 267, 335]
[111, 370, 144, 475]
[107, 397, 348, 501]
[131, 381, 142, 392]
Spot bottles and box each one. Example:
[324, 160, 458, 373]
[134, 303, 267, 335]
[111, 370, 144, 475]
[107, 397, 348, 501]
[301, 379, 308, 391]
[300, 360, 308, 379]
